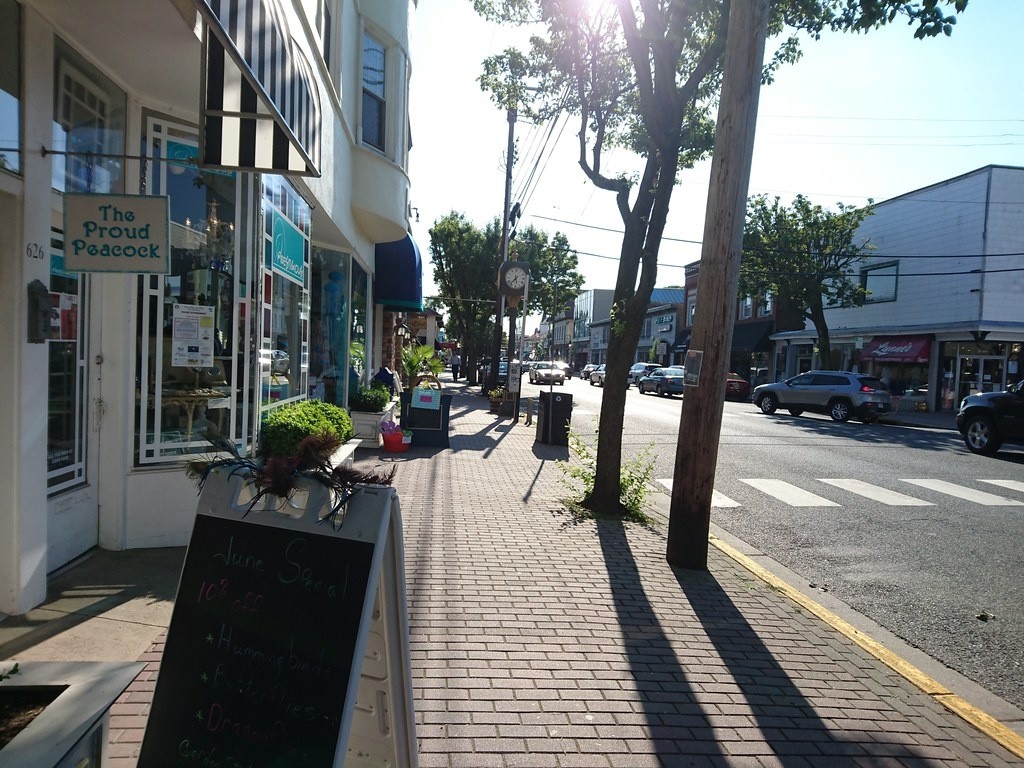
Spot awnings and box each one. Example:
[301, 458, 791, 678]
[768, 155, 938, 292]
[375, 231, 424, 313]
[196, 1, 321, 178]
[668, 323, 773, 353]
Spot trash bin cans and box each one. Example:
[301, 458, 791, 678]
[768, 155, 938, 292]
[534, 390, 573, 446]
[372, 366, 396, 400]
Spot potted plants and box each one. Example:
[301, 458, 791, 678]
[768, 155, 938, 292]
[487, 389, 514, 416]
[256, 398, 362, 479]
[350, 387, 396, 449]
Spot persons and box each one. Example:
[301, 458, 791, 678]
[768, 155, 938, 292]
[430, 350, 441, 378]
[450, 352, 460, 381]
[860, 336, 930, 362]
[889, 375, 906, 414]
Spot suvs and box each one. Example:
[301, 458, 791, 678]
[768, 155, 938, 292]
[589, 364, 607, 387]
[752, 369, 891, 423]
[630, 362, 662, 383]
[956, 379, 1024, 455]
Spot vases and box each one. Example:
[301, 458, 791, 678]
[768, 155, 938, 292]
[382, 432, 409, 452]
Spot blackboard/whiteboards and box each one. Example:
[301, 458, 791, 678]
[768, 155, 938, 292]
[134, 511, 376, 768]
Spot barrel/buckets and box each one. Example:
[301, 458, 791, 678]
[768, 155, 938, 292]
[382, 432, 411, 451]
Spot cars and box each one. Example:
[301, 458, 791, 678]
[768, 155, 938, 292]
[553, 362, 572, 379]
[580, 364, 599, 380]
[725, 373, 749, 401]
[487, 362, 510, 387]
[529, 361, 565, 385]
[522, 362, 529, 372]
[638, 368, 685, 397]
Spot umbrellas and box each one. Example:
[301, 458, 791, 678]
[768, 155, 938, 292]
[439, 340, 457, 357]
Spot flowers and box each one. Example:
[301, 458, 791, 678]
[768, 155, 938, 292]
[377, 420, 413, 437]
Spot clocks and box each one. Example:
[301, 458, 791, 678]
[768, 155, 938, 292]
[506, 267, 527, 290]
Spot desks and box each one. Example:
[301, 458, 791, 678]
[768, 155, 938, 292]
[141, 388, 227, 452]
